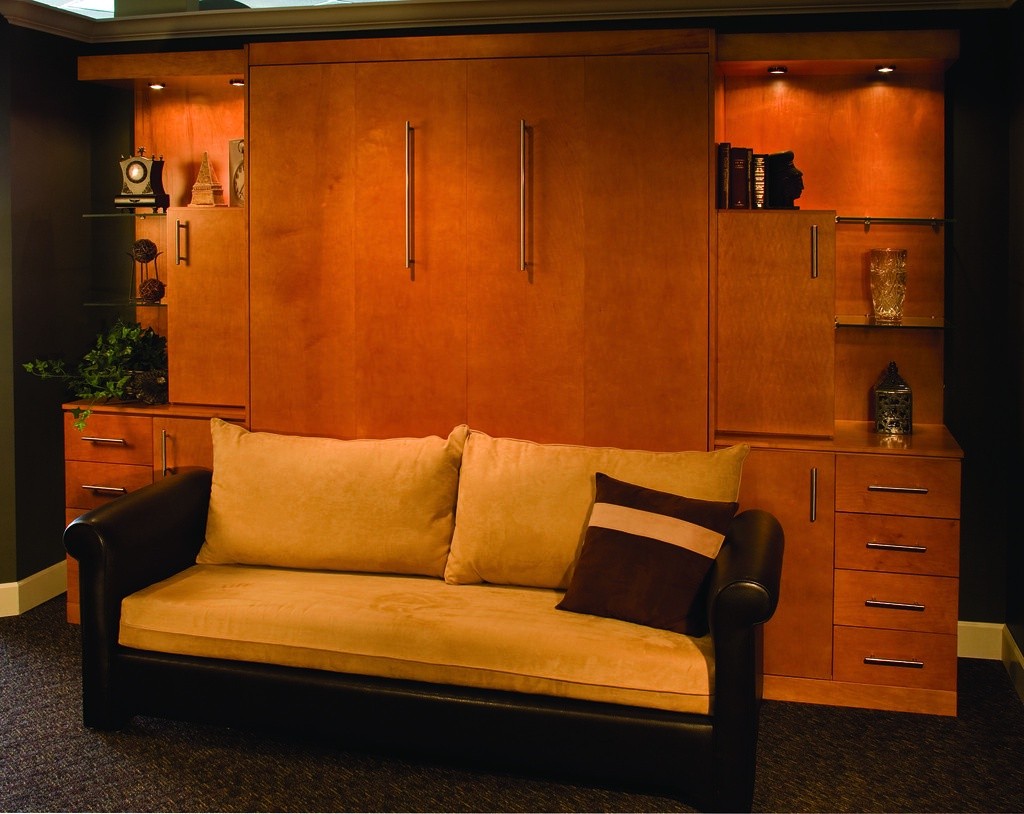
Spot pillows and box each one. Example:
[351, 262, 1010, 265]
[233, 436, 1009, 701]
[556, 471, 739, 636]
[195, 417, 469, 581]
[443, 429, 750, 591]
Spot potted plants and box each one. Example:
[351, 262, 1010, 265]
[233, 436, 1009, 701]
[22, 316, 170, 432]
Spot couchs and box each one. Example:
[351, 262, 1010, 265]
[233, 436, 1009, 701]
[61, 467, 785, 814]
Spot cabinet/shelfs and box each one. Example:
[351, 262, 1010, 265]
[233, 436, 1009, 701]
[64, 29, 958, 715]
